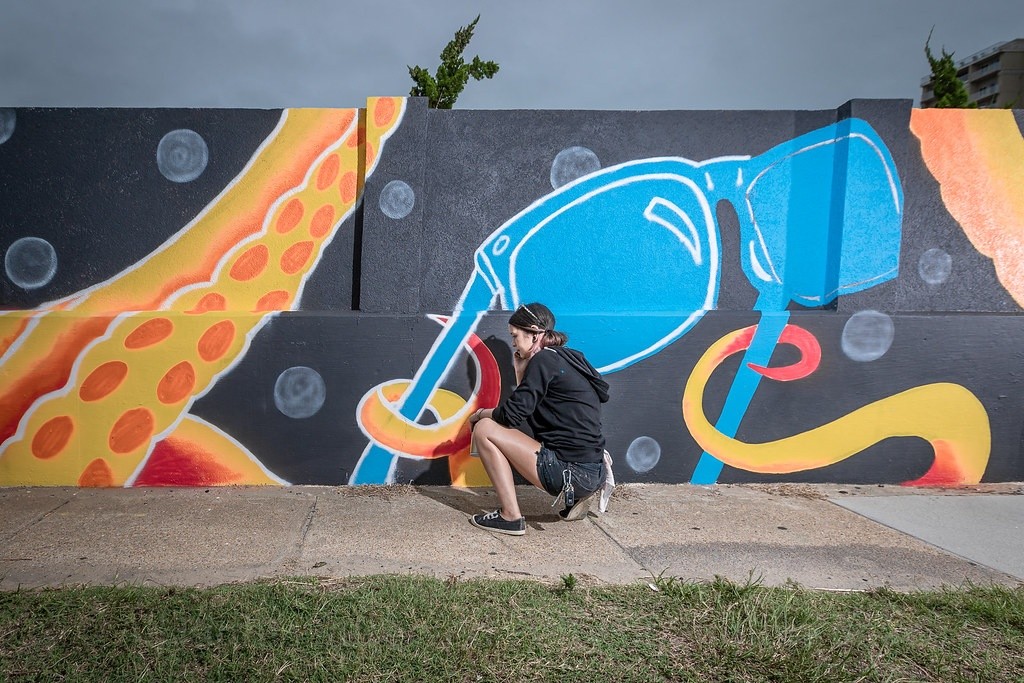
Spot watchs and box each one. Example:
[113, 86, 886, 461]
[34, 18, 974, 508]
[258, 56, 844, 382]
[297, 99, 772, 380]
[476, 408, 485, 420]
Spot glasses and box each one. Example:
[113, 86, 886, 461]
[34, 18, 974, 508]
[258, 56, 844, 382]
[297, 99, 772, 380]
[516, 303, 542, 324]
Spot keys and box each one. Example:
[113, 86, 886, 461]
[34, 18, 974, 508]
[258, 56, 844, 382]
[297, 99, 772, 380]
[551, 483, 575, 508]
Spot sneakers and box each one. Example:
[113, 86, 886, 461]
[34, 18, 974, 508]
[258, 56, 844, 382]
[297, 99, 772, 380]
[472, 507, 525, 535]
[558, 492, 596, 521]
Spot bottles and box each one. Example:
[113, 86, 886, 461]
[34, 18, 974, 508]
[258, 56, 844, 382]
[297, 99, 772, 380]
[470, 422, 480, 456]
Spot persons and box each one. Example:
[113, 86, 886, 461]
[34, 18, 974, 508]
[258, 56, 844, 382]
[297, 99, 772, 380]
[469, 302, 610, 536]
